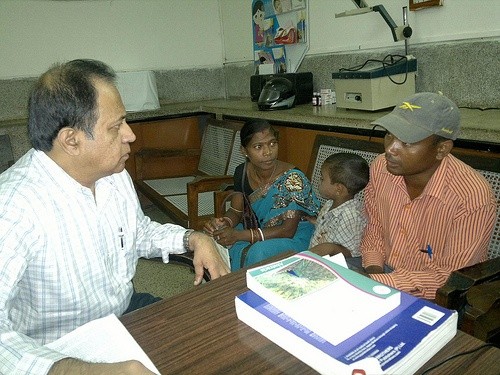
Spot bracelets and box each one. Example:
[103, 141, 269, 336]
[250, 228, 254, 244]
[183, 229, 194, 252]
[258, 228, 264, 241]
[223, 216, 233, 227]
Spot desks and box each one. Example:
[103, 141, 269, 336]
[120, 252, 499, 375]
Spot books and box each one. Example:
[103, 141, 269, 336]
[235, 284, 458, 375]
[246, 251, 401, 346]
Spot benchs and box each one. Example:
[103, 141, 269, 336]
[215, 134, 500, 348]
[133, 118, 279, 231]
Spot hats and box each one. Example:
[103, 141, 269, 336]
[371, 92, 459, 143]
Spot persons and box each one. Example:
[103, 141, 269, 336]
[203, 119, 320, 272]
[306, 152, 370, 259]
[0, 60, 231, 375]
[345, 92, 497, 301]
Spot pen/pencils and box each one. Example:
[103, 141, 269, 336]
[427, 244, 432, 259]
[120, 227, 123, 248]
